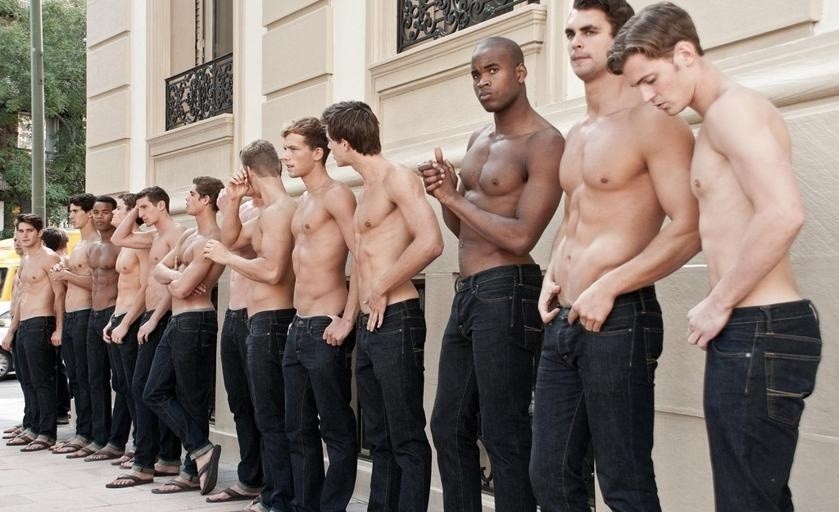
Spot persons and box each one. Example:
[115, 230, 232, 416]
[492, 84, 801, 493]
[529, 0, 700, 512]
[604, 3, 821, 512]
[417, 37, 566, 512]
[0, 102, 443, 511]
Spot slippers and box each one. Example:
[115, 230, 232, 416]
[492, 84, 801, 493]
[2, 416, 269, 512]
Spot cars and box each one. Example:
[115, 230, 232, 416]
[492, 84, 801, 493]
[0, 226, 82, 387]
